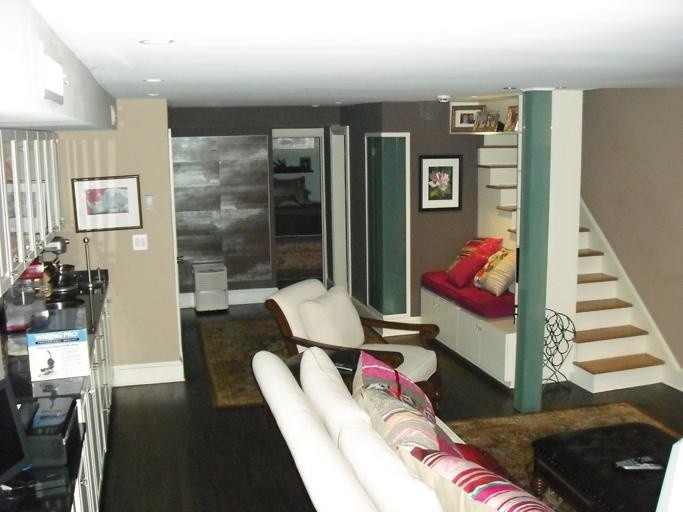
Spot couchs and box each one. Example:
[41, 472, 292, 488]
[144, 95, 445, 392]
[250, 349, 556, 512]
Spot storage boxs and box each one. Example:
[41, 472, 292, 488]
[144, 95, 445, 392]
[25, 307, 91, 384]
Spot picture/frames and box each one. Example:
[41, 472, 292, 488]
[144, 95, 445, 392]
[273, 138, 314, 149]
[451, 105, 519, 132]
[300, 157, 312, 170]
[71, 175, 143, 233]
[419, 155, 463, 212]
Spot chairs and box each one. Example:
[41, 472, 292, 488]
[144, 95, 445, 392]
[264, 278, 440, 411]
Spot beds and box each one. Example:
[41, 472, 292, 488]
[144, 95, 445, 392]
[272, 176, 320, 235]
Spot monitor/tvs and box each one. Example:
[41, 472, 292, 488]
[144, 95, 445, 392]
[0, 378, 32, 494]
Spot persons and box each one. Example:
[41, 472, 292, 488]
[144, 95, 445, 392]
[467, 113, 474, 124]
[460, 114, 471, 124]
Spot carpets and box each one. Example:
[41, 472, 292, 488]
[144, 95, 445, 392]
[199, 318, 440, 407]
[447, 401, 683, 511]
[273, 237, 322, 280]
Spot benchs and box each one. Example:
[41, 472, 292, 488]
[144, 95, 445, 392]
[528, 423, 680, 512]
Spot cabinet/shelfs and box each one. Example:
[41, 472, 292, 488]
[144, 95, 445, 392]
[420, 287, 517, 391]
[192, 263, 229, 313]
[0, 130, 63, 298]
[68, 297, 111, 512]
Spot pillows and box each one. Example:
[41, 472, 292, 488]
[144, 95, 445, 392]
[299, 284, 365, 350]
[349, 348, 556, 512]
[274, 195, 302, 209]
[447, 237, 520, 297]
